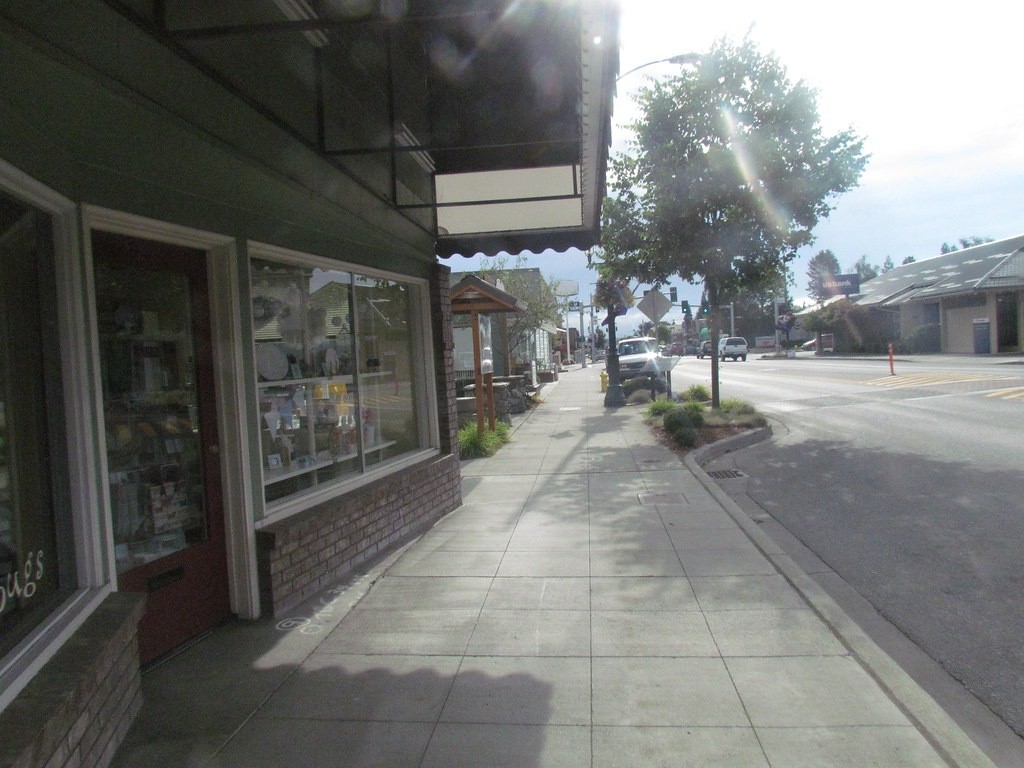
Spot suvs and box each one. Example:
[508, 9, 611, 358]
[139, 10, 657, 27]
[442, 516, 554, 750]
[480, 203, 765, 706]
[617, 337, 662, 382]
[718, 337, 748, 362]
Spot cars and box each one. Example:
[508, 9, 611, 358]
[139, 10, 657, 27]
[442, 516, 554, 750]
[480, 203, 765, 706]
[595, 349, 607, 361]
[801, 333, 833, 349]
[658, 339, 712, 359]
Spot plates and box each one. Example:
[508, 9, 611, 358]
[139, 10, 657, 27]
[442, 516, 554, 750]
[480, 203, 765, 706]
[325, 348, 340, 374]
[257, 345, 289, 381]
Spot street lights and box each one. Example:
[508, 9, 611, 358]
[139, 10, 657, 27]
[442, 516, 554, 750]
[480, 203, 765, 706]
[604, 53, 702, 407]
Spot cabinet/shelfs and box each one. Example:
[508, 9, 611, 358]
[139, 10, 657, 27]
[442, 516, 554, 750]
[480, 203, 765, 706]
[258, 368, 398, 487]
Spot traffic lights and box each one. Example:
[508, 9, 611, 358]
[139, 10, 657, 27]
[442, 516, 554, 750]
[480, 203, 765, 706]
[701, 307, 710, 315]
[681, 301, 689, 313]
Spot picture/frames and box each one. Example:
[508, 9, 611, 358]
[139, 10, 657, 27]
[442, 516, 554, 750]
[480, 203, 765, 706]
[267, 453, 282, 471]
